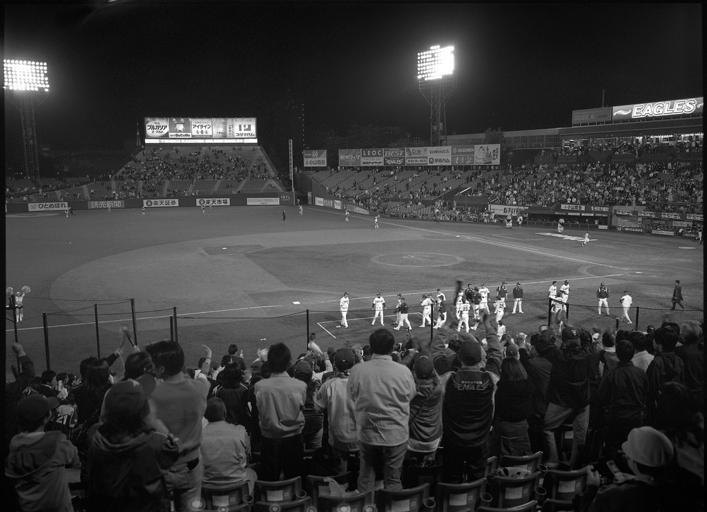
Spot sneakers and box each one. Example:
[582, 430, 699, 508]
[511, 311, 524, 314]
[616, 317, 632, 325]
[341, 322, 349, 328]
[456, 324, 477, 333]
[419, 322, 442, 330]
[371, 322, 412, 331]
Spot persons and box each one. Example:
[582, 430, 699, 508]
[6, 145, 279, 219]
[332, 133, 703, 232]
[299, 203, 303, 215]
[281, 210, 286, 224]
[5, 280, 706, 512]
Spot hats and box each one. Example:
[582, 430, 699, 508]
[601, 283, 605, 286]
[565, 280, 569, 283]
[621, 426, 674, 467]
[503, 280, 508, 284]
[345, 292, 350, 295]
[376, 292, 380, 295]
[459, 343, 481, 362]
[104, 373, 156, 418]
[15, 395, 58, 422]
[518, 282, 521, 285]
